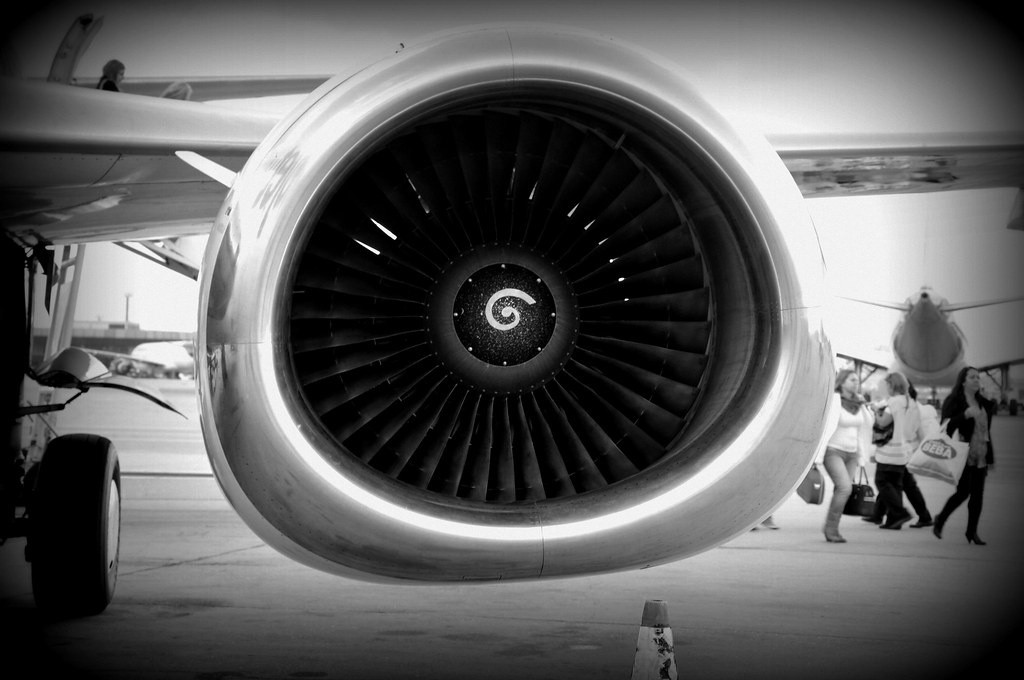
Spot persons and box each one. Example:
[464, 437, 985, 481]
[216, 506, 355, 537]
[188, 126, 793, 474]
[750, 367, 934, 544]
[932, 366, 999, 545]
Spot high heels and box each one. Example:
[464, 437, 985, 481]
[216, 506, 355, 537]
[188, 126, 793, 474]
[965, 530, 987, 545]
[932, 514, 943, 539]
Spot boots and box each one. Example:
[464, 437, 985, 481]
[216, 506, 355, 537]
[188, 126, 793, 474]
[824, 512, 847, 543]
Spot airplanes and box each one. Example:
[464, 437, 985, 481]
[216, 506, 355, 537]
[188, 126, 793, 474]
[0, 0, 1024, 680]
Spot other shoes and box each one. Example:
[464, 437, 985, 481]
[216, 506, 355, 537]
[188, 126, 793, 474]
[763, 518, 779, 529]
[862, 514, 882, 524]
[909, 519, 932, 527]
[880, 511, 910, 529]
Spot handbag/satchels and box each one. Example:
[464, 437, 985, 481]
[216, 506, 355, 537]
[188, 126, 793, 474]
[871, 405, 893, 446]
[797, 464, 825, 504]
[908, 418, 969, 485]
[843, 468, 874, 517]
